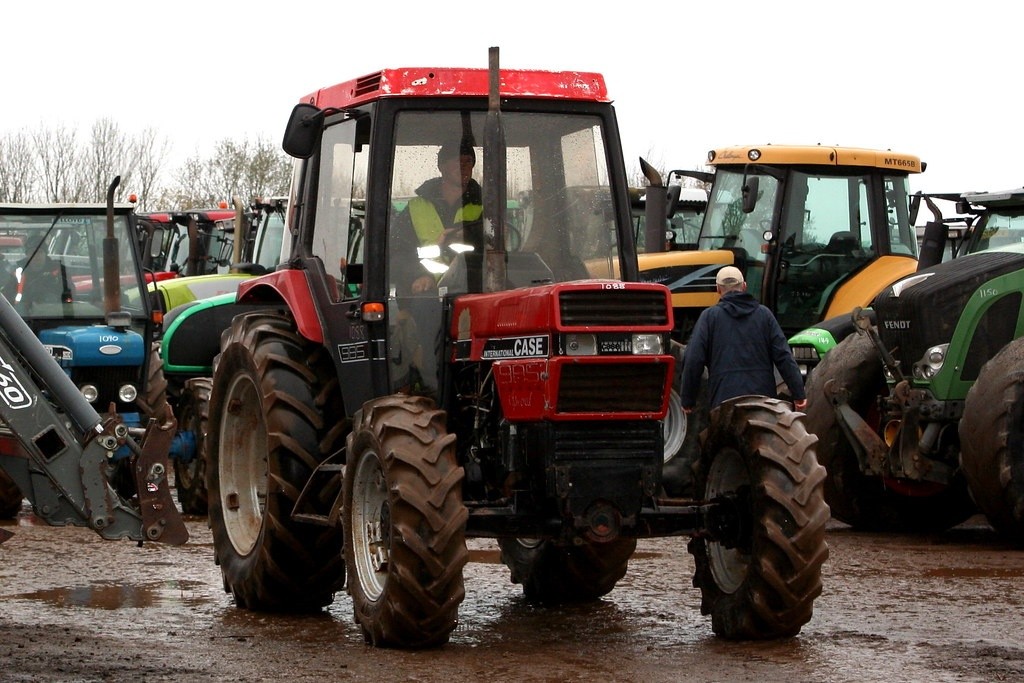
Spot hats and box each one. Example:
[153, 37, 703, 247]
[716, 265, 745, 286]
[438, 138, 477, 162]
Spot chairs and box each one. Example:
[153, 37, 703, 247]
[798, 230, 862, 291]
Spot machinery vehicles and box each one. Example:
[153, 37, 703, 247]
[200, 44, 834, 655]
[1, 146, 1023, 558]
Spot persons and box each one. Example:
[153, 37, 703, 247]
[391, 138, 483, 295]
[12, 235, 77, 303]
[681, 265, 807, 413]
